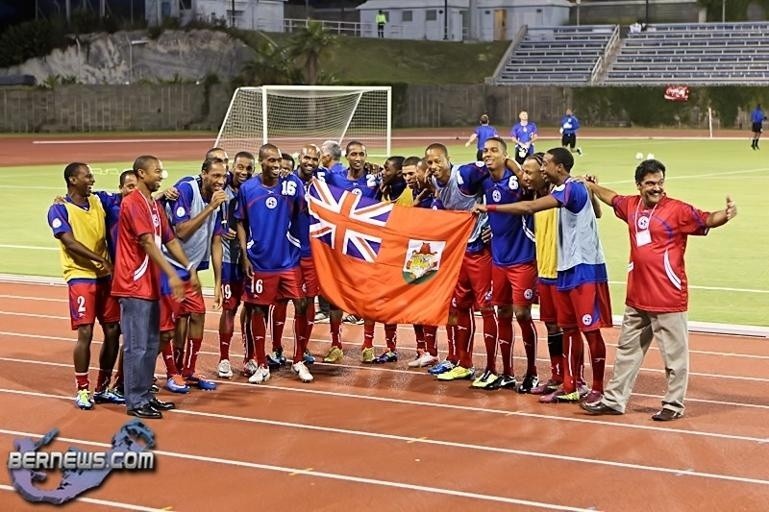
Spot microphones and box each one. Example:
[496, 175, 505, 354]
[219, 189, 228, 228]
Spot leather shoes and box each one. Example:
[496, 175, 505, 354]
[580, 400, 624, 415]
[651, 407, 685, 422]
[126, 399, 175, 420]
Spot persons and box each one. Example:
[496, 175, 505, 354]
[563, 160, 738, 423]
[750, 103, 768, 150]
[375, 10, 387, 38]
[464, 114, 501, 162]
[511, 110, 538, 164]
[559, 107, 582, 157]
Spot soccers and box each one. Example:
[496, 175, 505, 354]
[635, 153, 655, 160]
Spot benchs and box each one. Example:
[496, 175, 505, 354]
[486, 20, 769, 86]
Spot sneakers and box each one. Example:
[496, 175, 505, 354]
[576, 147, 582, 156]
[76, 346, 624, 409]
